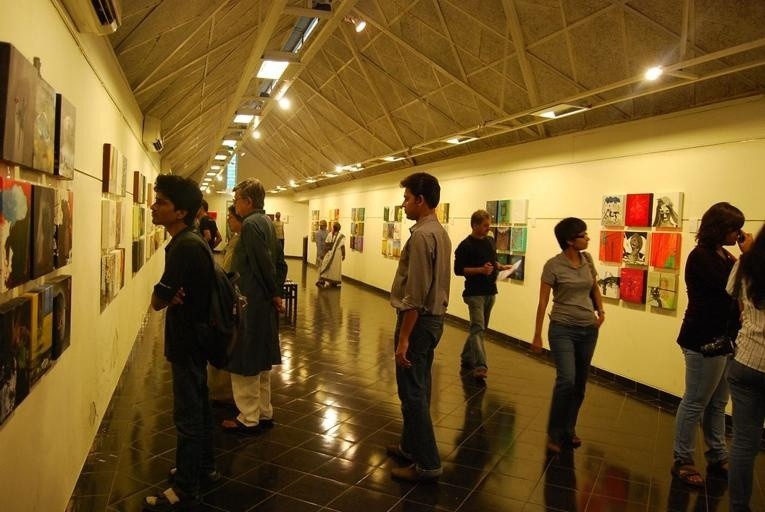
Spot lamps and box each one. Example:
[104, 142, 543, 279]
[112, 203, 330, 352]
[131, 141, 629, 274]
[342, 16, 368, 33]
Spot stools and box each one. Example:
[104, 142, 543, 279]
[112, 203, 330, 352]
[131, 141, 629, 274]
[281, 279, 297, 322]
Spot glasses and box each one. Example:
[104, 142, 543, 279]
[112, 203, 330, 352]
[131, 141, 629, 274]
[576, 232, 588, 239]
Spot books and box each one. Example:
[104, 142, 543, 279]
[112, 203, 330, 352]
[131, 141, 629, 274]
[498, 260, 522, 278]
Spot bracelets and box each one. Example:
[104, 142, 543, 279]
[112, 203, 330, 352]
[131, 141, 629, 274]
[597, 310, 605, 316]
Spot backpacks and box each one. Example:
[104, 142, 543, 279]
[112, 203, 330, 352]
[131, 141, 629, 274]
[169, 232, 249, 369]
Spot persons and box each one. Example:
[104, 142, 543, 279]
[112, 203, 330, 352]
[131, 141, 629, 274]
[654, 197, 677, 226]
[453, 210, 515, 383]
[313, 219, 331, 279]
[722, 224, 765, 511]
[316, 222, 346, 288]
[625, 234, 645, 261]
[387, 173, 450, 482]
[671, 201, 754, 484]
[531, 217, 606, 459]
[145, 170, 288, 509]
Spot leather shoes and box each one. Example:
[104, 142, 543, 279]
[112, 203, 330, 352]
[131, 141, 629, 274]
[387, 443, 399, 456]
[391, 467, 438, 481]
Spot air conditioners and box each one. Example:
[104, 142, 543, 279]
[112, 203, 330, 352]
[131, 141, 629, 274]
[61, 0, 124, 36]
[143, 114, 164, 153]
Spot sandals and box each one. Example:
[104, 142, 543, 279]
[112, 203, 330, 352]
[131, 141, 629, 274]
[166, 466, 221, 487]
[221, 418, 254, 433]
[671, 464, 706, 487]
[143, 483, 202, 512]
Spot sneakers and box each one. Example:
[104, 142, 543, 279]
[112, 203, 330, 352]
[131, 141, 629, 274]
[475, 368, 487, 377]
